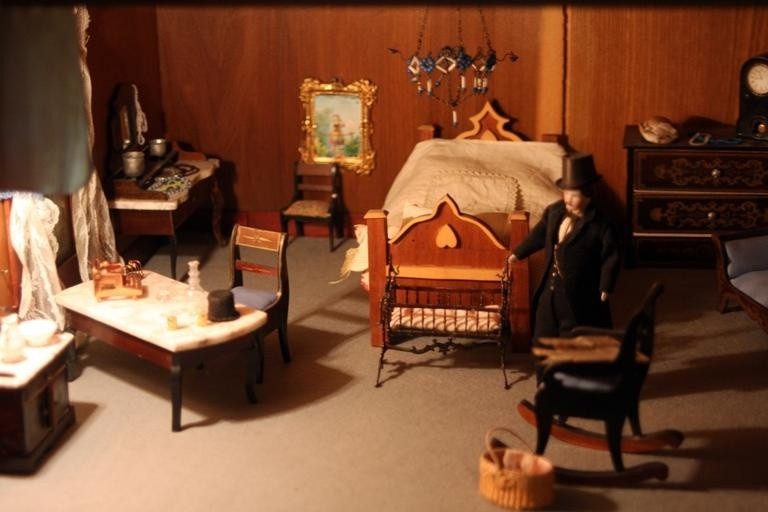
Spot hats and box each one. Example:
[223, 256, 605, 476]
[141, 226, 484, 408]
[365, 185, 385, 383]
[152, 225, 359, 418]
[555, 153, 603, 191]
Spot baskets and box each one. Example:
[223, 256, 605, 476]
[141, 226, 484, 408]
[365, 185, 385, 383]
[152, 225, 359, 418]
[477, 427, 555, 512]
